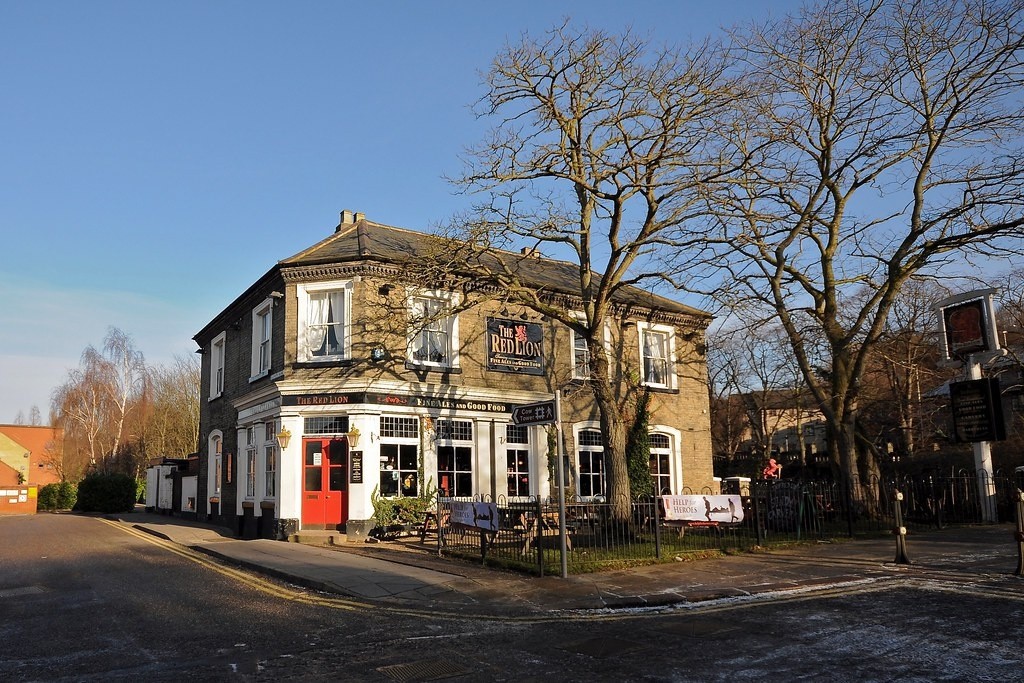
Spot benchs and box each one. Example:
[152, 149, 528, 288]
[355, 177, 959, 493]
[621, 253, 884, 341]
[417, 522, 602, 556]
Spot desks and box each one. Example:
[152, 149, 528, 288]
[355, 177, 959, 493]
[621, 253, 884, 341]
[509, 502, 600, 540]
[443, 508, 573, 556]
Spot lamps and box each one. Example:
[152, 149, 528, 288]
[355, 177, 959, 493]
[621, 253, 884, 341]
[195, 349, 207, 355]
[379, 284, 395, 295]
[227, 324, 241, 331]
[344, 423, 361, 451]
[266, 291, 285, 300]
[275, 425, 292, 451]
[512, 307, 528, 320]
[492, 307, 508, 318]
[683, 332, 700, 341]
[532, 313, 548, 323]
[623, 321, 634, 328]
